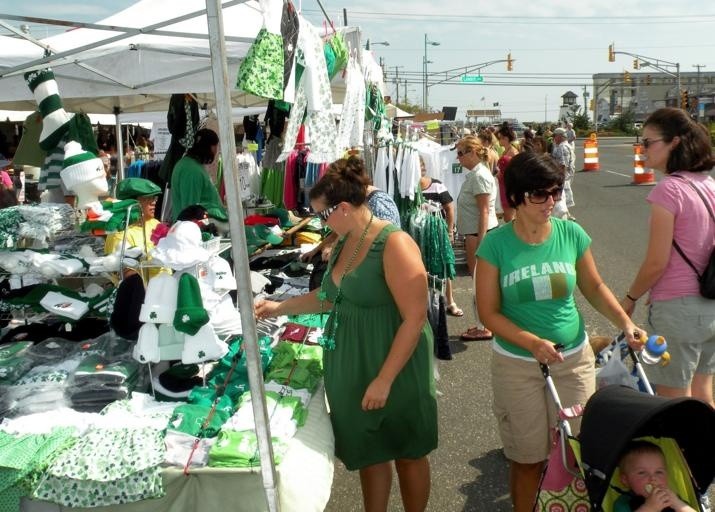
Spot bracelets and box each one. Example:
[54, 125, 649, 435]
[626, 295, 637, 301]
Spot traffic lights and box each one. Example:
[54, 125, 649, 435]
[589, 99, 595, 110]
[681, 90, 689, 110]
[507, 53, 512, 71]
[633, 57, 640, 70]
[624, 70, 631, 85]
[647, 74, 651, 86]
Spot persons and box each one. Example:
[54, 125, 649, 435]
[480, 120, 576, 221]
[613, 440, 699, 512]
[330, 158, 400, 228]
[619, 108, 715, 413]
[252, 156, 437, 512]
[456, 135, 498, 341]
[124, 136, 153, 161]
[104, 176, 168, 288]
[419, 157, 463, 316]
[474, 152, 648, 512]
[2, 158, 27, 203]
[169, 128, 226, 222]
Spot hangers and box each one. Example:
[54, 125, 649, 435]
[296, 0, 338, 37]
[425, 271, 448, 312]
[413, 199, 444, 228]
[232, 141, 310, 154]
[379, 115, 419, 151]
[132, 150, 167, 161]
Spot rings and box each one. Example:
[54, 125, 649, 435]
[544, 358, 548, 364]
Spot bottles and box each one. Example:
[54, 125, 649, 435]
[638, 334, 672, 369]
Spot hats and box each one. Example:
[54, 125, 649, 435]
[208, 292, 243, 336]
[32, 253, 89, 276]
[550, 127, 569, 138]
[114, 176, 164, 201]
[173, 272, 210, 336]
[206, 255, 238, 291]
[132, 324, 161, 364]
[157, 323, 186, 361]
[244, 206, 303, 258]
[181, 322, 229, 365]
[151, 363, 204, 399]
[175, 205, 209, 223]
[109, 272, 146, 341]
[58, 139, 108, 191]
[149, 219, 213, 271]
[137, 273, 178, 324]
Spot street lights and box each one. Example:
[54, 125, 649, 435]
[364, 30, 441, 114]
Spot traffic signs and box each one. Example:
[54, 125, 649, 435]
[462, 76, 483, 81]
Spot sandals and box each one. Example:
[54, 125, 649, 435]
[459, 325, 493, 341]
[445, 302, 464, 317]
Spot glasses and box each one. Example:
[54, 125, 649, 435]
[640, 135, 673, 153]
[457, 148, 472, 157]
[313, 201, 342, 224]
[522, 185, 563, 204]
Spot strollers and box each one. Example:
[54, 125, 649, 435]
[535, 329, 715, 512]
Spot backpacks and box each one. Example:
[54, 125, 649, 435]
[666, 174, 715, 302]
